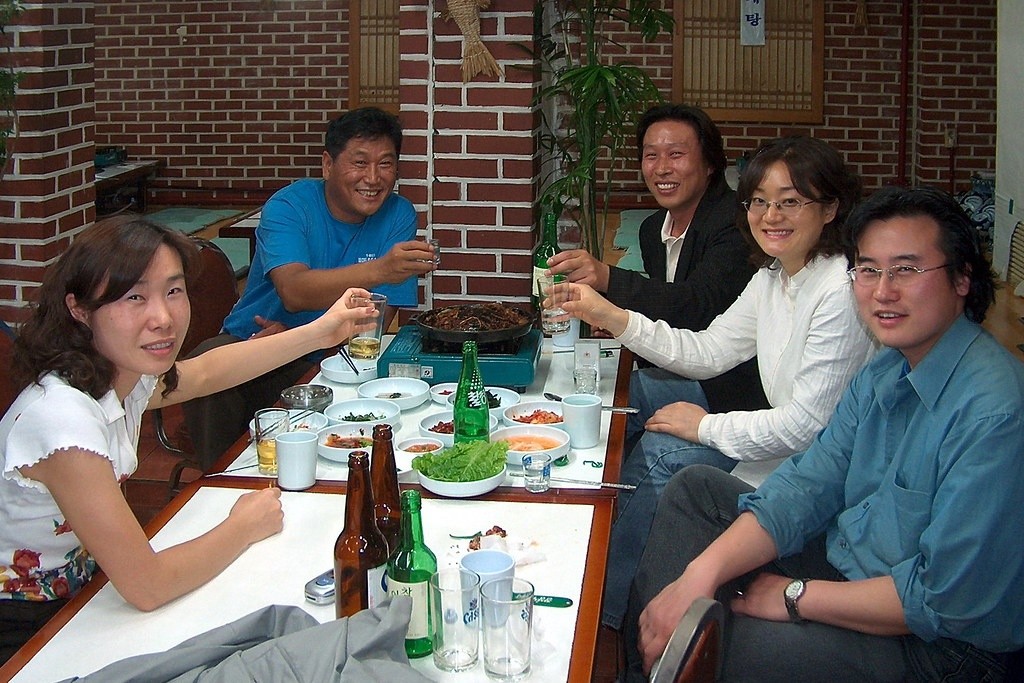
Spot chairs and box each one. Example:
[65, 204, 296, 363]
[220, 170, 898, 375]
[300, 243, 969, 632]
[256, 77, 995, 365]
[147, 237, 242, 487]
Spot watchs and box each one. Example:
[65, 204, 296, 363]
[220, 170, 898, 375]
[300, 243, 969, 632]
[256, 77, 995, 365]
[784, 577, 812, 625]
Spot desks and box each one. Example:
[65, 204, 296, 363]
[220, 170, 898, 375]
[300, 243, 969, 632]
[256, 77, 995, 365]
[218, 204, 271, 267]
[94, 159, 161, 215]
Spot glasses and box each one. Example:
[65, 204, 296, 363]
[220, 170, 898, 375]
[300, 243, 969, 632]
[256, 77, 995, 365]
[846, 264, 952, 288]
[742, 196, 825, 215]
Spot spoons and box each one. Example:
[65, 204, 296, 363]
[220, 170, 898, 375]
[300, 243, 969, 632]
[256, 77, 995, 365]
[544, 393, 639, 413]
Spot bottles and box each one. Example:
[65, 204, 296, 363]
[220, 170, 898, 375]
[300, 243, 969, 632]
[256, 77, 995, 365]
[387, 488, 442, 658]
[530, 213, 564, 311]
[333, 451, 389, 620]
[454, 341, 490, 444]
[360, 425, 413, 614]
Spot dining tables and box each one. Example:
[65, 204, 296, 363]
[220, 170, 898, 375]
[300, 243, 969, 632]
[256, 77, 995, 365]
[0, 336, 635, 683]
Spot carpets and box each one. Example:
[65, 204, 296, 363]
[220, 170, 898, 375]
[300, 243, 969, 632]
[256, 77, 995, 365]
[145, 208, 244, 236]
[209, 234, 249, 270]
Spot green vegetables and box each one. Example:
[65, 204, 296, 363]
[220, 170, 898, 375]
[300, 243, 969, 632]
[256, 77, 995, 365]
[485, 389, 503, 408]
[458, 305, 495, 331]
[339, 411, 387, 421]
[390, 393, 401, 398]
[412, 440, 510, 481]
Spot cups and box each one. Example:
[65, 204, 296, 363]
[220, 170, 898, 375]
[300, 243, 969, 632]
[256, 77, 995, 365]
[275, 431, 317, 492]
[573, 369, 597, 395]
[460, 550, 515, 626]
[348, 293, 387, 362]
[253, 407, 292, 476]
[553, 317, 582, 347]
[522, 452, 551, 493]
[422, 239, 441, 265]
[562, 395, 603, 448]
[431, 568, 481, 671]
[537, 274, 571, 335]
[480, 576, 534, 680]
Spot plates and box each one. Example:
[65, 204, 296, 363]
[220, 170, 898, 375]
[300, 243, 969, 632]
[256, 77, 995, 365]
[248, 376, 569, 498]
[320, 355, 380, 384]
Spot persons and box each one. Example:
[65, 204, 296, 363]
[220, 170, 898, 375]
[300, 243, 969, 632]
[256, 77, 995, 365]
[545, 103, 772, 459]
[624, 186, 1024, 683]
[543, 134, 888, 630]
[0, 214, 379, 669]
[178, 107, 438, 473]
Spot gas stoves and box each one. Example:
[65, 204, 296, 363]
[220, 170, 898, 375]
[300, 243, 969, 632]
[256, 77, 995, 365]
[377, 324, 543, 394]
[95, 145, 127, 167]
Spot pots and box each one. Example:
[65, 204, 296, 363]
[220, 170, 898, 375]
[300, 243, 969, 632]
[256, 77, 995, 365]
[409, 304, 539, 345]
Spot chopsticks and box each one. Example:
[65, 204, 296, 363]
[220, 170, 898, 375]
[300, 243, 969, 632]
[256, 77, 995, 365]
[251, 405, 320, 444]
[336, 346, 359, 376]
